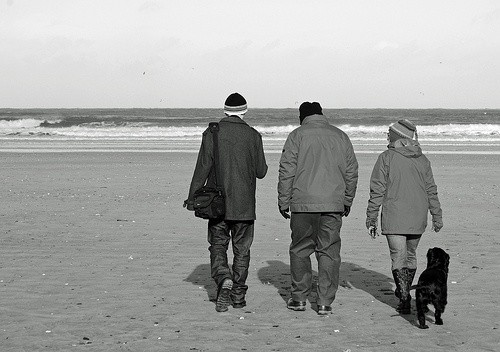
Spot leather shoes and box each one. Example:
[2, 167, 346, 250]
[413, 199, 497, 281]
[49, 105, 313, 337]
[288, 298, 306, 310]
[318, 305, 334, 313]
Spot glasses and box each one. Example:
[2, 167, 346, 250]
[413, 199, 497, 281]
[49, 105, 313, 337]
[387, 133, 390, 137]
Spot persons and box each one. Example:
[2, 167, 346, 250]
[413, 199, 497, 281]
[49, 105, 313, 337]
[366, 119, 443, 314]
[278, 102, 359, 313]
[183, 92, 268, 312]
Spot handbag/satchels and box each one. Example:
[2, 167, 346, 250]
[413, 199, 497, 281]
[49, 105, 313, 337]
[194, 184, 225, 220]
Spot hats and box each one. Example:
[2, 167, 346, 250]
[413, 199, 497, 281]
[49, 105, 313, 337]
[299, 102, 323, 125]
[224, 93, 247, 115]
[389, 119, 418, 143]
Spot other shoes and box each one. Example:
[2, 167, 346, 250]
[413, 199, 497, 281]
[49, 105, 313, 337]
[232, 299, 246, 307]
[216, 277, 233, 312]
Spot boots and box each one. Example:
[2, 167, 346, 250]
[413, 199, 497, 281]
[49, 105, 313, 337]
[393, 267, 411, 314]
[395, 269, 416, 301]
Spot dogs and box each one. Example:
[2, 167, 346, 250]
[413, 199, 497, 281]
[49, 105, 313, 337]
[404, 244, 451, 330]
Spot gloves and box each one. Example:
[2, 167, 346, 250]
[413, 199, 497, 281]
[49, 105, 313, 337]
[368, 225, 379, 238]
[340, 205, 350, 216]
[279, 206, 290, 219]
[187, 205, 195, 211]
[431, 221, 443, 232]
